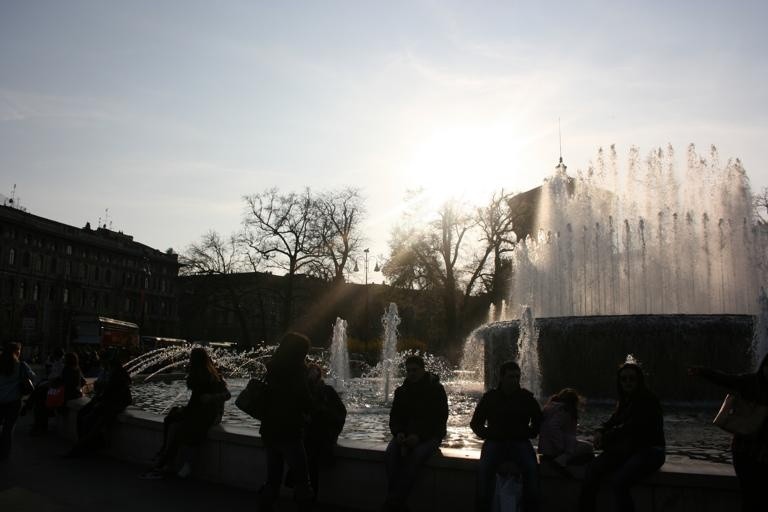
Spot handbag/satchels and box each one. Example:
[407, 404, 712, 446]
[712, 392, 768, 436]
[46, 385, 64, 407]
[23, 377, 36, 394]
[235, 379, 279, 423]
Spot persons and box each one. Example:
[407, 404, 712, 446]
[247, 333, 320, 502]
[1, 340, 35, 464]
[60, 359, 134, 459]
[576, 364, 666, 511]
[136, 348, 232, 479]
[538, 386, 593, 468]
[31, 341, 144, 377]
[673, 352, 766, 511]
[28, 351, 85, 437]
[380, 357, 448, 510]
[469, 360, 543, 511]
[20, 370, 63, 433]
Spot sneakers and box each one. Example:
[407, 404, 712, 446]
[20, 405, 48, 438]
[137, 466, 172, 481]
